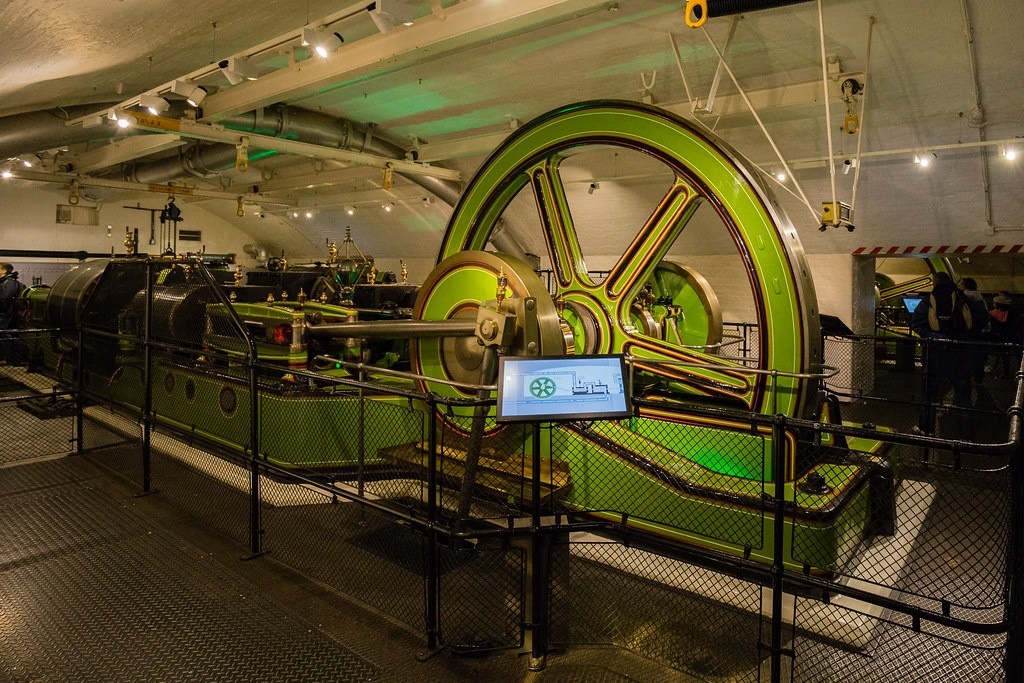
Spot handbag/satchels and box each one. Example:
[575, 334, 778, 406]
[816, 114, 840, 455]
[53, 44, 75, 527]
[951, 287, 989, 341]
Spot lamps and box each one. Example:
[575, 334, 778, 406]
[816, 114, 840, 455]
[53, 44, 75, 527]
[920, 152, 937, 166]
[587, 183, 597, 194]
[170, 80, 208, 107]
[108, 108, 136, 128]
[218, 57, 259, 85]
[138, 94, 171, 115]
[422, 198, 432, 208]
[301, 27, 345, 57]
[366, 0, 410, 35]
[348, 205, 357, 214]
[385, 201, 394, 212]
[24, 158, 41, 168]
[840, 158, 856, 175]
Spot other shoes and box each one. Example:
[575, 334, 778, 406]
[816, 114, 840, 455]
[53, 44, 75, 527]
[911, 425, 934, 437]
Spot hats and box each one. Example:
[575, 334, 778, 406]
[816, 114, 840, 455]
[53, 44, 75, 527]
[993, 295, 1009, 305]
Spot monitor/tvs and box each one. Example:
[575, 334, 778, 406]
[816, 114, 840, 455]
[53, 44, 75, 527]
[496, 352, 634, 423]
[901, 297, 924, 314]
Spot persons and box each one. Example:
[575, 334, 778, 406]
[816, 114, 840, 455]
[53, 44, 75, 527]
[908, 270, 989, 437]
[0, 261, 21, 330]
[958, 276, 989, 413]
[989, 295, 1015, 383]
